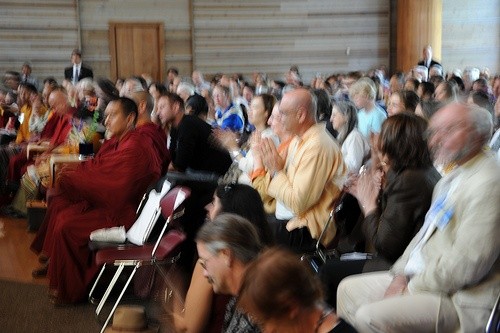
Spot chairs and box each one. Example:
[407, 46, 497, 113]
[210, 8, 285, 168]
[88, 176, 191, 333]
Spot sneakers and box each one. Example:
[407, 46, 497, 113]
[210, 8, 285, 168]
[90, 226, 127, 244]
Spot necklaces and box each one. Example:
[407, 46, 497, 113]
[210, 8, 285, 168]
[313, 308, 331, 333]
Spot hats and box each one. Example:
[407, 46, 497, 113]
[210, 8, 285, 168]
[105, 304, 161, 333]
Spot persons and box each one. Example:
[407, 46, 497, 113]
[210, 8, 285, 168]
[0, 60, 500, 333]
[63, 52, 94, 81]
[417, 46, 442, 68]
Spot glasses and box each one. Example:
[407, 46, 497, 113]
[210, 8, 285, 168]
[199, 253, 220, 270]
[387, 100, 405, 108]
